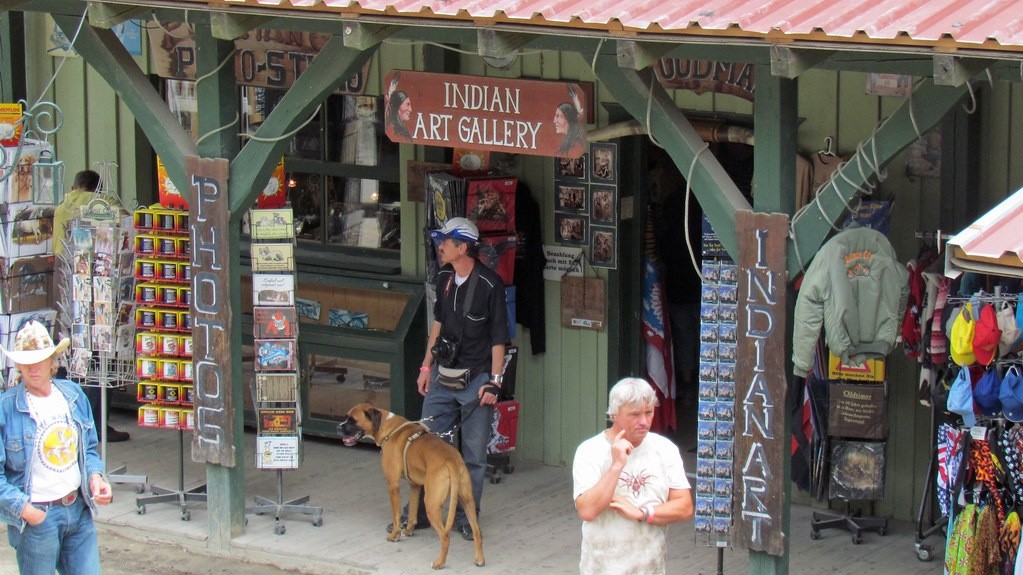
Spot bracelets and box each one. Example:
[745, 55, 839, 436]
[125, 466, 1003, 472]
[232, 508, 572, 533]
[419, 367, 431, 372]
[490, 375, 503, 383]
[640, 505, 653, 524]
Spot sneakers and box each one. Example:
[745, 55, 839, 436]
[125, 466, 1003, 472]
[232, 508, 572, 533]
[387, 515, 430, 533]
[458, 524, 474, 540]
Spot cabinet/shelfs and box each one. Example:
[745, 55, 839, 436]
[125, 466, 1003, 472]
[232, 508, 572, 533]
[247, 201, 325, 536]
[238, 262, 428, 454]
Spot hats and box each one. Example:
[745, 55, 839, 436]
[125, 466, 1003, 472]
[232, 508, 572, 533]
[947, 366, 976, 427]
[972, 305, 1001, 366]
[430, 216, 479, 246]
[950, 303, 976, 366]
[997, 301, 1019, 357]
[0, 320, 70, 364]
[975, 370, 1001, 416]
[998, 365, 1023, 423]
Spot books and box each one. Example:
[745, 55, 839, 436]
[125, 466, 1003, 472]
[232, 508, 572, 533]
[252, 207, 301, 472]
[296, 297, 321, 320]
[0, 103, 66, 388]
[329, 308, 368, 326]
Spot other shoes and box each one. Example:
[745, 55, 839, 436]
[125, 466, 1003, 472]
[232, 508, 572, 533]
[106, 426, 130, 441]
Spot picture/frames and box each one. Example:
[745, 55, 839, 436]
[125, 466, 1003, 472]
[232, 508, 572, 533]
[552, 142, 618, 270]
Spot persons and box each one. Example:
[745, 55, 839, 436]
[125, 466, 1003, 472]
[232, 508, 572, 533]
[54, 169, 131, 442]
[387, 218, 507, 532]
[594, 232, 611, 260]
[559, 158, 583, 176]
[572, 377, 695, 575]
[0, 320, 113, 575]
[559, 189, 580, 208]
[559, 218, 581, 238]
[596, 154, 611, 180]
[592, 191, 611, 221]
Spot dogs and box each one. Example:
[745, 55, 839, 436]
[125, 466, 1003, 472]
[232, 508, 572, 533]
[337, 402, 485, 570]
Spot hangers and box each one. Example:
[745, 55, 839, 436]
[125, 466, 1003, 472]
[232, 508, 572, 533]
[801, 135, 849, 173]
[905, 229, 986, 281]
[822, 210, 898, 256]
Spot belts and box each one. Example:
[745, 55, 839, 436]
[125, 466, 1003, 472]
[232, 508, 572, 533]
[31, 489, 79, 506]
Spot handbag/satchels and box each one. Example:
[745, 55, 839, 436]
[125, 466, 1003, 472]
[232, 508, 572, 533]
[437, 365, 470, 390]
